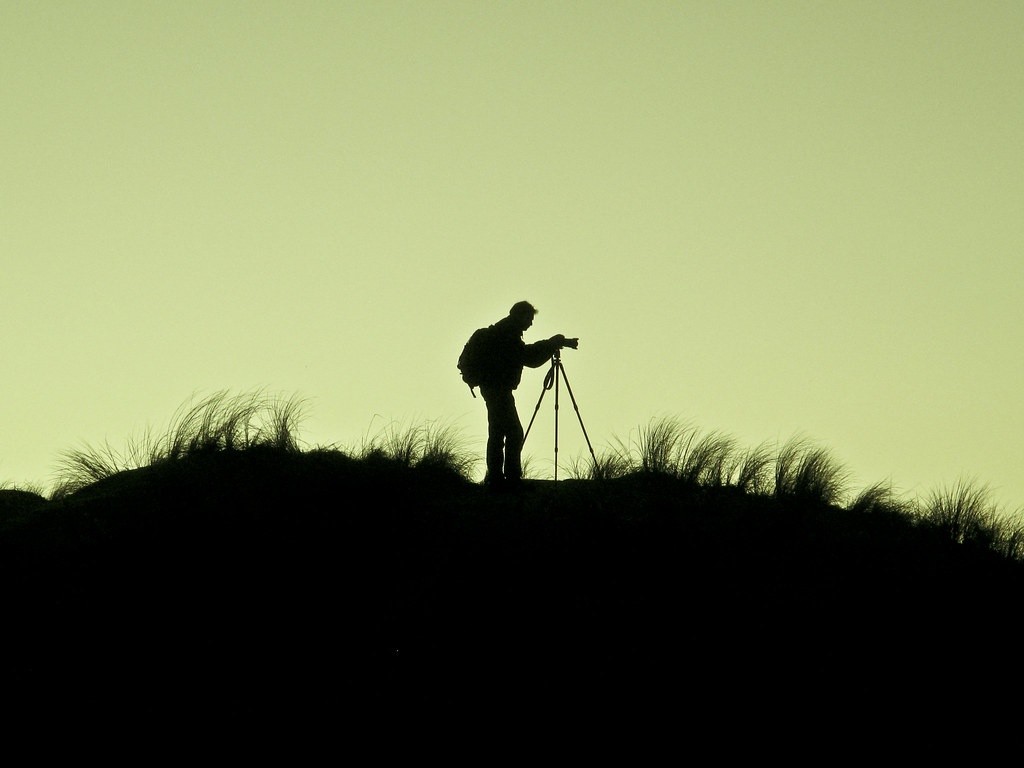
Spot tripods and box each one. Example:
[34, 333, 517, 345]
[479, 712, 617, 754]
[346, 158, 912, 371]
[521, 350, 603, 486]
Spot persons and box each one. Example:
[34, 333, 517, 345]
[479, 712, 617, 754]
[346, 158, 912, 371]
[479, 301, 568, 480]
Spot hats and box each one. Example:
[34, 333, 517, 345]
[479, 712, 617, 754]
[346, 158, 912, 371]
[509, 301, 538, 315]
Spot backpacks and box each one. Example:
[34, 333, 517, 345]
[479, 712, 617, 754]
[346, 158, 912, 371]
[457, 324, 512, 397]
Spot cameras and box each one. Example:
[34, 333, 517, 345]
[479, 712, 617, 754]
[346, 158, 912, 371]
[562, 338, 579, 350]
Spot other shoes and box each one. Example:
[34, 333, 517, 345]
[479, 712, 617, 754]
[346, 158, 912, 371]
[485, 475, 505, 484]
[505, 473, 521, 480]
